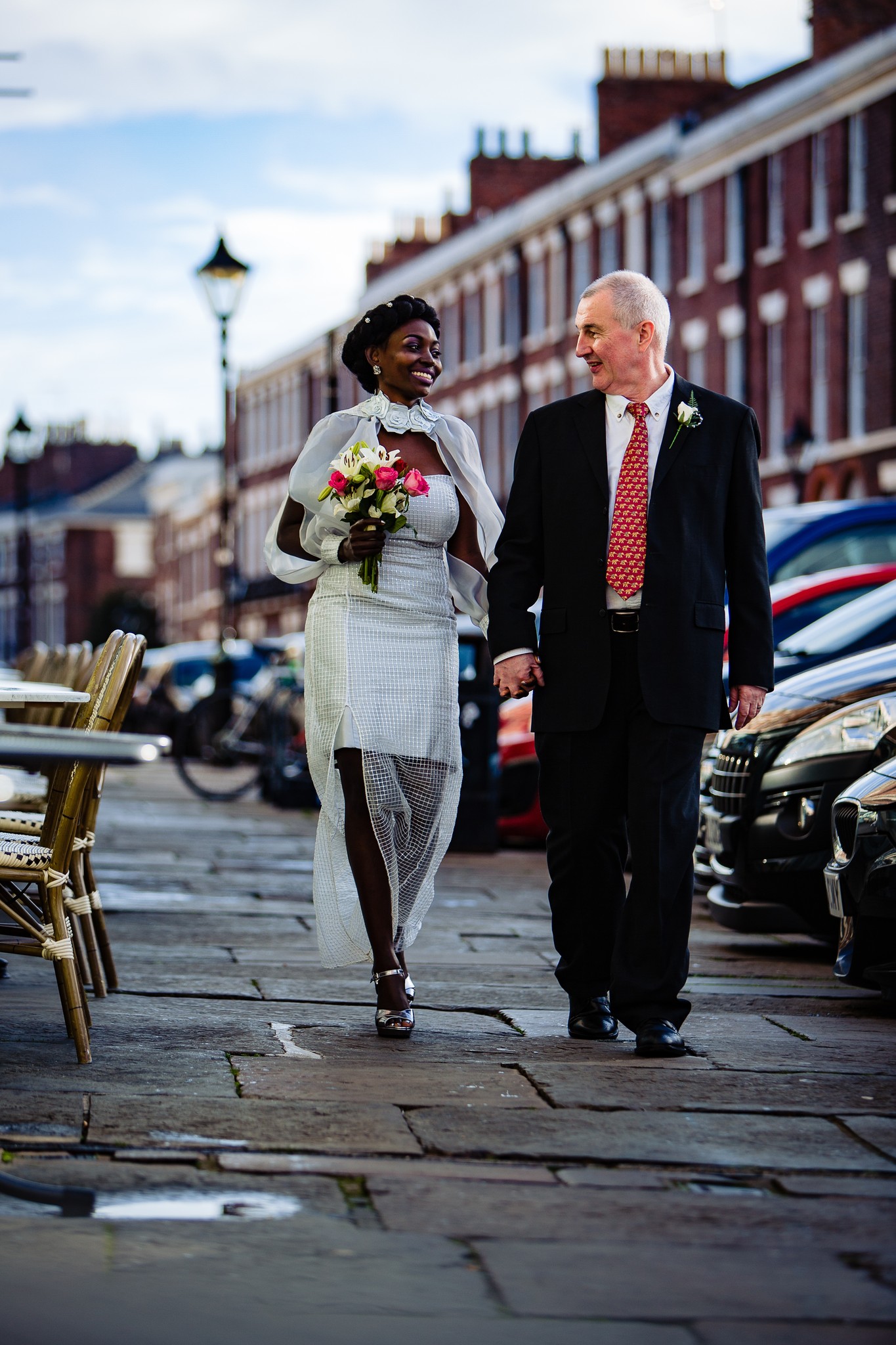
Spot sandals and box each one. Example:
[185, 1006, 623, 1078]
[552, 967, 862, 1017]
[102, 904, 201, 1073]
[368, 965, 415, 1040]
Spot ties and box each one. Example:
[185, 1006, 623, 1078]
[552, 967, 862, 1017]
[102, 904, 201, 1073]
[605, 401, 650, 602]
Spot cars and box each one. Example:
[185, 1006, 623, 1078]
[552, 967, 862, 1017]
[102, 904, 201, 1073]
[140, 485, 895, 994]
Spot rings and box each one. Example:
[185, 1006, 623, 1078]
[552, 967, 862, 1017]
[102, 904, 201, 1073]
[757, 705, 763, 710]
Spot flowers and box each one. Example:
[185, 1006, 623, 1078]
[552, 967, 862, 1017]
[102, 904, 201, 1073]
[317, 439, 429, 594]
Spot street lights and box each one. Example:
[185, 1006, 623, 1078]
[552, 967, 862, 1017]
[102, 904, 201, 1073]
[200, 234, 261, 732]
[6, 410, 41, 657]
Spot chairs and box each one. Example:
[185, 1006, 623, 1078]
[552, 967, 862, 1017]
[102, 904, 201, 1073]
[0, 626, 173, 1064]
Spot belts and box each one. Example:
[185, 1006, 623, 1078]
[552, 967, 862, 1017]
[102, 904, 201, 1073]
[606, 609, 643, 635]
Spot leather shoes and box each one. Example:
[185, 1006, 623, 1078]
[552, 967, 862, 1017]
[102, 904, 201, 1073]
[635, 1018, 686, 1058]
[567, 996, 619, 1040]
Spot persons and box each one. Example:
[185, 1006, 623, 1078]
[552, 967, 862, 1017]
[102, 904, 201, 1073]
[263, 291, 538, 1038]
[485, 272, 775, 1057]
